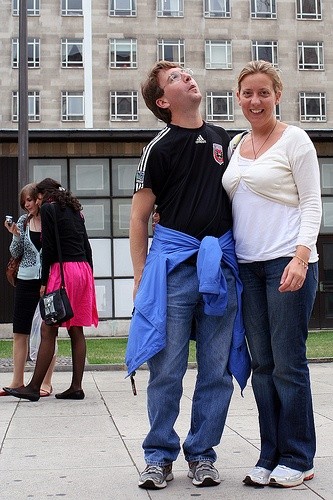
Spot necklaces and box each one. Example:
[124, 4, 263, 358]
[250, 119, 277, 159]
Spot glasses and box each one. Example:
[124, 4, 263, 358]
[162, 68, 194, 89]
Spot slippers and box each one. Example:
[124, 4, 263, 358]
[0, 385, 25, 396]
[40, 385, 52, 396]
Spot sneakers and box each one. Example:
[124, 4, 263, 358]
[268, 464, 314, 487]
[138, 463, 173, 490]
[243, 467, 271, 486]
[187, 461, 221, 486]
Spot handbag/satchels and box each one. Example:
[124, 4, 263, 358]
[39, 287, 74, 326]
[6, 215, 26, 287]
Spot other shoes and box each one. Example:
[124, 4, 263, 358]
[55, 390, 85, 399]
[3, 387, 40, 401]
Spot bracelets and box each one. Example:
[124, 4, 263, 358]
[293, 255, 309, 269]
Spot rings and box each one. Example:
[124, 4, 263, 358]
[297, 282, 301, 287]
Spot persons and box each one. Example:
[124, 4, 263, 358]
[0, 183, 58, 398]
[222, 60, 321, 489]
[124, 58, 252, 490]
[0, 177, 98, 401]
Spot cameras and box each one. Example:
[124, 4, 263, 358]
[5, 215, 13, 223]
